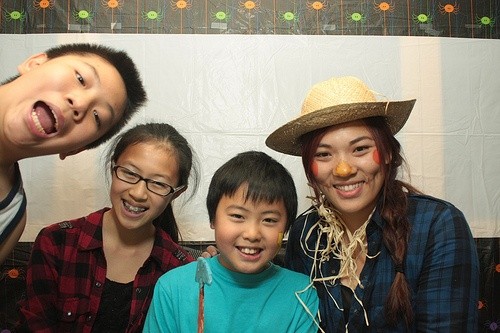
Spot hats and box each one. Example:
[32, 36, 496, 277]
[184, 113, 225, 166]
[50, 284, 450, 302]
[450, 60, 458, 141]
[263, 75, 416, 156]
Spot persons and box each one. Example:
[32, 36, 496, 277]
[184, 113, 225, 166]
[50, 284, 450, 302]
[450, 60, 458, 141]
[0, 43, 147, 261]
[201, 76, 480, 333]
[15, 124, 195, 333]
[143, 151, 319, 333]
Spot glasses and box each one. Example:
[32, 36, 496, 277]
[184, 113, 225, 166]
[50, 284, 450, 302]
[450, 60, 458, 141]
[112, 160, 187, 198]
